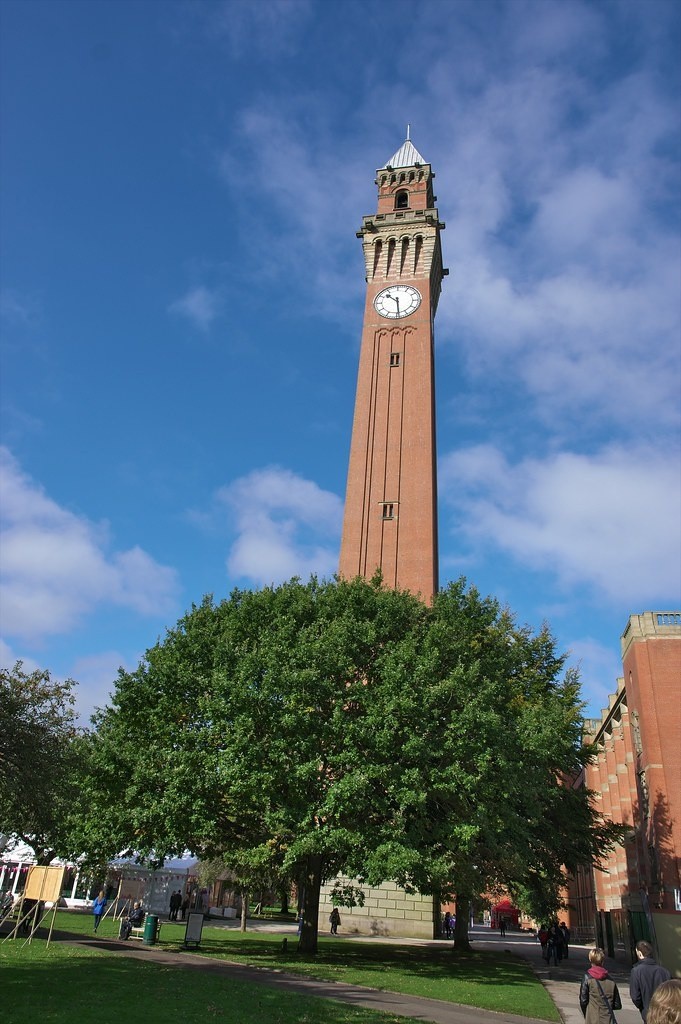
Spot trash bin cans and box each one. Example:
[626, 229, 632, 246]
[142, 915, 159, 946]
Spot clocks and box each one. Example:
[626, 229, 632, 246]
[372, 283, 423, 320]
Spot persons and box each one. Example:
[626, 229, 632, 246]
[0, 890, 681, 1024]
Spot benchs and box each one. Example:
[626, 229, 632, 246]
[118, 915, 162, 944]
